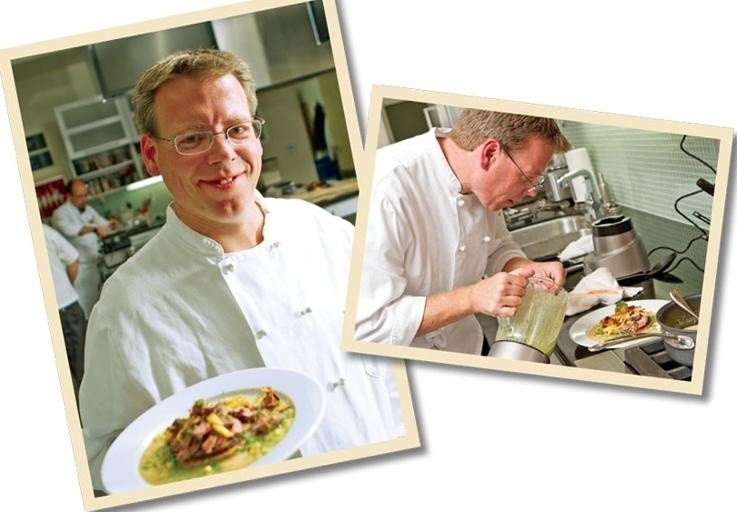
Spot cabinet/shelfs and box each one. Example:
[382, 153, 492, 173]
[53, 88, 160, 201]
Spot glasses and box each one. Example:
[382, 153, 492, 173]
[504, 148, 543, 194]
[156, 114, 267, 157]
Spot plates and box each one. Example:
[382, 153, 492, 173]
[568, 299, 672, 349]
[100, 365, 327, 495]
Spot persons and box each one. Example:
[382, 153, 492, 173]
[354, 106, 573, 356]
[49, 178, 120, 324]
[74, 46, 409, 497]
[43, 223, 86, 386]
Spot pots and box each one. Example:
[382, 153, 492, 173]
[588, 292, 701, 367]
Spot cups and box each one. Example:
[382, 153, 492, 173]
[486, 276, 569, 363]
